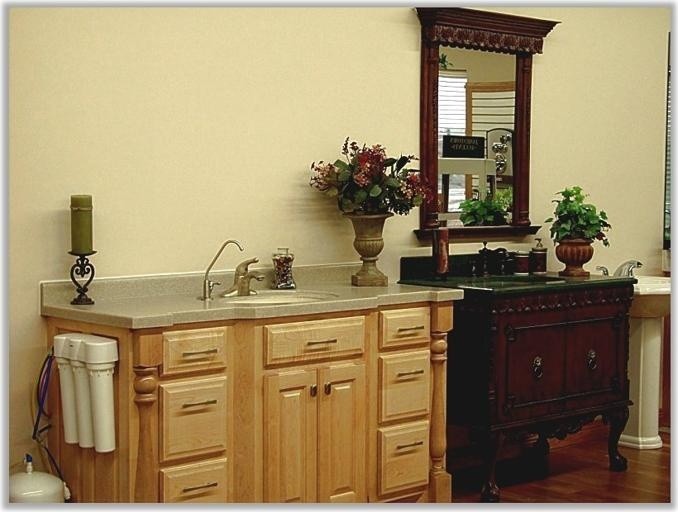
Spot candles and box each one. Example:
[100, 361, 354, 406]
[70, 193, 96, 255]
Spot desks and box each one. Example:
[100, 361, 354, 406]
[437, 156, 498, 227]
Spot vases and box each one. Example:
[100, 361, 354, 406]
[343, 208, 398, 288]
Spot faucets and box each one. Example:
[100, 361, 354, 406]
[612, 259, 643, 277]
[477, 239, 510, 276]
[200, 239, 244, 301]
[220, 257, 267, 297]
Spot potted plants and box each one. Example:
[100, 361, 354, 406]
[548, 184, 610, 281]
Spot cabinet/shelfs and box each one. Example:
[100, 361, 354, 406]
[447, 284, 633, 443]
[370, 304, 438, 504]
[237, 317, 380, 501]
[42, 313, 242, 504]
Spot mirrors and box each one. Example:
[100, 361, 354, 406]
[408, 7, 562, 243]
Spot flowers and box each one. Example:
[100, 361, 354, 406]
[310, 142, 432, 215]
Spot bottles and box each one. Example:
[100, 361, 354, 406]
[269, 247, 296, 290]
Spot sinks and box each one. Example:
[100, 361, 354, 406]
[224, 289, 340, 303]
[468, 275, 566, 286]
[629, 275, 671, 318]
